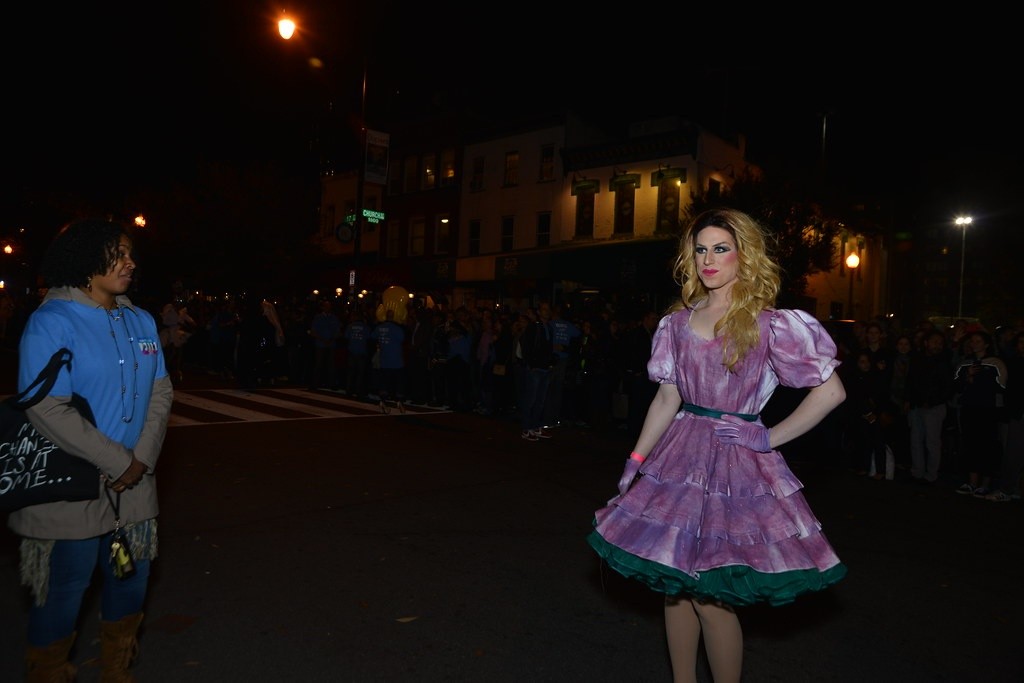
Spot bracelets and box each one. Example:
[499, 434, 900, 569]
[630, 452, 645, 463]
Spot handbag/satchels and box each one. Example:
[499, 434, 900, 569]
[0, 348, 99, 514]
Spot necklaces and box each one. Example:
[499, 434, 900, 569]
[106, 304, 138, 423]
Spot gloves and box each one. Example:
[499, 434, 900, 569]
[714, 414, 771, 453]
[617, 459, 641, 496]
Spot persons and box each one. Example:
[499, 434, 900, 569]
[17, 221, 173, 683]
[157, 289, 657, 441]
[841, 319, 1024, 502]
[0, 291, 15, 341]
[587, 209, 847, 683]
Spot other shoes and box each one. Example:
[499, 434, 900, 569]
[985, 489, 1021, 502]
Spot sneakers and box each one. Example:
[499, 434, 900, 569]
[973, 486, 988, 499]
[530, 427, 554, 439]
[521, 430, 540, 441]
[955, 482, 978, 494]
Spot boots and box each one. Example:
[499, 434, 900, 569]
[99, 610, 142, 683]
[25, 631, 77, 683]
